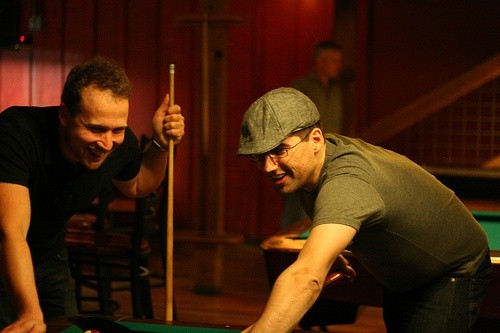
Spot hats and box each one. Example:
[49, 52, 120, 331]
[236, 87, 320, 155]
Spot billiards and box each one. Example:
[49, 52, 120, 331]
[84, 330, 100, 333]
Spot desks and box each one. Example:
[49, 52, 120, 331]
[260, 210, 500, 333]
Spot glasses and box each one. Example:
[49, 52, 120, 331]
[247, 128, 316, 161]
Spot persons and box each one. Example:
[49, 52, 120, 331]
[0, 62, 181, 333]
[283, 41, 347, 230]
[236, 85, 492, 333]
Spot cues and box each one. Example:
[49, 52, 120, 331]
[165, 64, 175, 321]
[240, 272, 347, 333]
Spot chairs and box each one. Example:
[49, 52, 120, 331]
[69, 172, 168, 320]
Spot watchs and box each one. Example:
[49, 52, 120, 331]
[150, 137, 170, 152]
